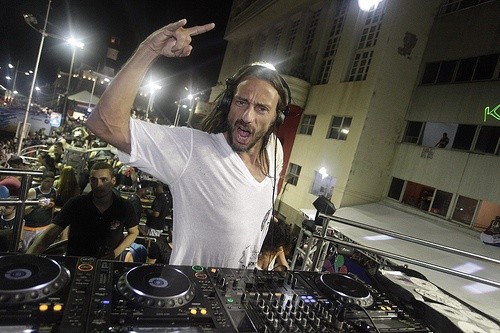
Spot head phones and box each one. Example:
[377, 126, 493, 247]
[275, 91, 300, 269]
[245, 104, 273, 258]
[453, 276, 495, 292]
[216, 65, 291, 127]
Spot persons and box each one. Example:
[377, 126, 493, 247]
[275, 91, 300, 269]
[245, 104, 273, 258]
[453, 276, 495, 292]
[297, 228, 373, 274]
[148, 224, 173, 265]
[256, 221, 290, 271]
[0, 94, 159, 265]
[271, 210, 280, 223]
[85, 19, 290, 271]
[144, 182, 171, 237]
